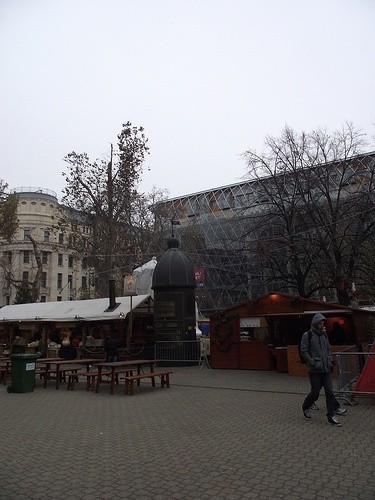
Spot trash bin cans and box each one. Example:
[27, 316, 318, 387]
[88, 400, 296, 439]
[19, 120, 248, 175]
[7, 353, 40, 393]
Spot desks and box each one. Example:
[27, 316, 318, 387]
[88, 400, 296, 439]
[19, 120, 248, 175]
[4, 358, 65, 386]
[92, 359, 159, 395]
[43, 358, 108, 390]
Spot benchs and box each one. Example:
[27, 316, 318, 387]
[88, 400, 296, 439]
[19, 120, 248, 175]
[0, 365, 107, 391]
[120, 370, 175, 396]
[82, 369, 137, 392]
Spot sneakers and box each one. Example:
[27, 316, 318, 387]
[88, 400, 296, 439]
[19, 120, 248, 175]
[311, 402, 319, 410]
[304, 409, 311, 418]
[335, 408, 347, 415]
[328, 415, 342, 426]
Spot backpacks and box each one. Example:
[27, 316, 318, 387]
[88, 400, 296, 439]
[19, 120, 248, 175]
[298, 331, 327, 364]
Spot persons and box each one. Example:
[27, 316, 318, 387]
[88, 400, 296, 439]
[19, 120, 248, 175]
[11, 335, 25, 345]
[104, 336, 121, 362]
[301, 313, 348, 428]
